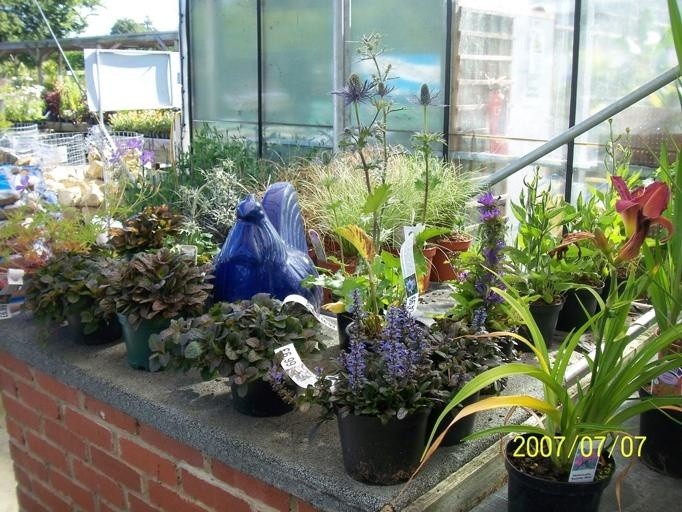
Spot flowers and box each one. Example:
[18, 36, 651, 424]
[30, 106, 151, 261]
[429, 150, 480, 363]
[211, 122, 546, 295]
[391, 171, 682, 508]
[324, 181, 508, 411]
[299, 22, 482, 227]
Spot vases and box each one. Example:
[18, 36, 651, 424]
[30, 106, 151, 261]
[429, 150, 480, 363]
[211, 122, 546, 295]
[503, 431, 611, 512]
[327, 404, 427, 486]
[427, 385, 484, 447]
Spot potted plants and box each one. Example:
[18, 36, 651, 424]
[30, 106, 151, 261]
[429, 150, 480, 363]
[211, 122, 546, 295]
[6, 52, 336, 414]
[634, 135, 682, 479]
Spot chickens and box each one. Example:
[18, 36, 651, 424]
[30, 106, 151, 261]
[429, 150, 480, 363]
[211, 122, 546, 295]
[211, 181, 323, 313]
